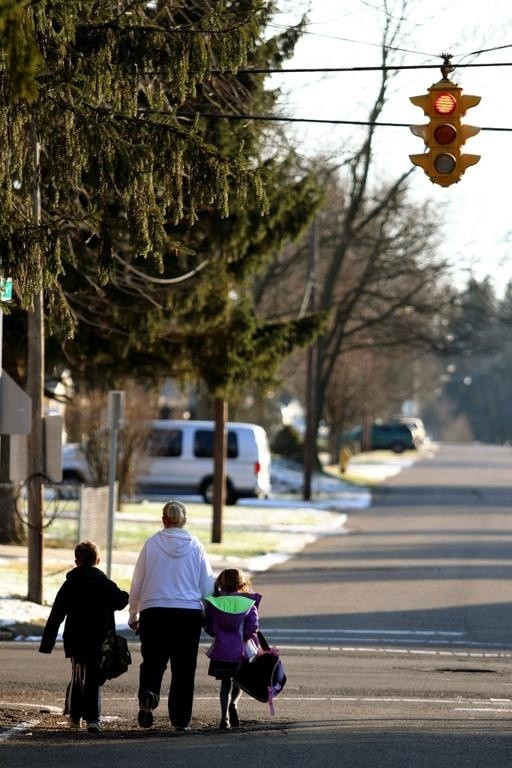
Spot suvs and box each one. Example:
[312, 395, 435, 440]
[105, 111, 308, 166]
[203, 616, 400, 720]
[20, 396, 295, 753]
[337, 418, 426, 453]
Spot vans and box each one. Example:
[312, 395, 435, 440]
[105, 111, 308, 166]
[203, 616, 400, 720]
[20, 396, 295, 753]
[62, 418, 272, 503]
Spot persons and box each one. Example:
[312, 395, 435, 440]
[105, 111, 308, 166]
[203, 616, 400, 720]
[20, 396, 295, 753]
[201, 567, 263, 728]
[129, 497, 215, 735]
[38, 537, 129, 735]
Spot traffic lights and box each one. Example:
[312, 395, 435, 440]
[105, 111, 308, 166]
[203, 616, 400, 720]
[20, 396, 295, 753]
[428, 85, 461, 186]
[458, 88, 483, 177]
[408, 88, 431, 179]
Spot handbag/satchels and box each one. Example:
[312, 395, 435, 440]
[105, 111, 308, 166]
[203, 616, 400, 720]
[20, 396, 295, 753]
[98, 633, 132, 686]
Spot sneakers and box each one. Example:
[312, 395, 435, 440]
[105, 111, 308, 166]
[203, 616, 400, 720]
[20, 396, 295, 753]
[175, 723, 191, 733]
[220, 718, 231, 729]
[138, 691, 155, 728]
[229, 703, 240, 726]
[70, 717, 83, 730]
[87, 722, 103, 732]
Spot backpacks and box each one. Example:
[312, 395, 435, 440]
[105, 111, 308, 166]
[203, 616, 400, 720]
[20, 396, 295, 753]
[231, 632, 286, 703]
